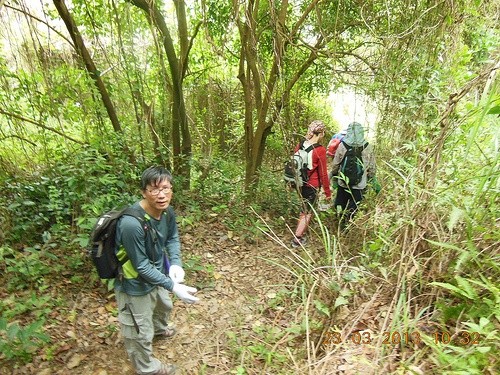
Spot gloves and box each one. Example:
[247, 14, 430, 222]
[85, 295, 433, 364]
[169, 264, 186, 284]
[172, 283, 198, 303]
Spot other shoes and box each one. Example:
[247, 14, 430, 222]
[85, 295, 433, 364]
[291, 236, 311, 248]
[152, 364, 177, 375]
[154, 328, 176, 338]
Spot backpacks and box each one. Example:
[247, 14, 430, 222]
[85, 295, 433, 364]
[92, 205, 159, 285]
[341, 140, 369, 186]
[326, 135, 344, 157]
[283, 142, 321, 189]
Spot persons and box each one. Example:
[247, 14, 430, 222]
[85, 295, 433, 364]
[287, 120, 331, 248]
[328, 122, 378, 234]
[113, 164, 200, 375]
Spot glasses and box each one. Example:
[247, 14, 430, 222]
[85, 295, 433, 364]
[144, 184, 173, 196]
